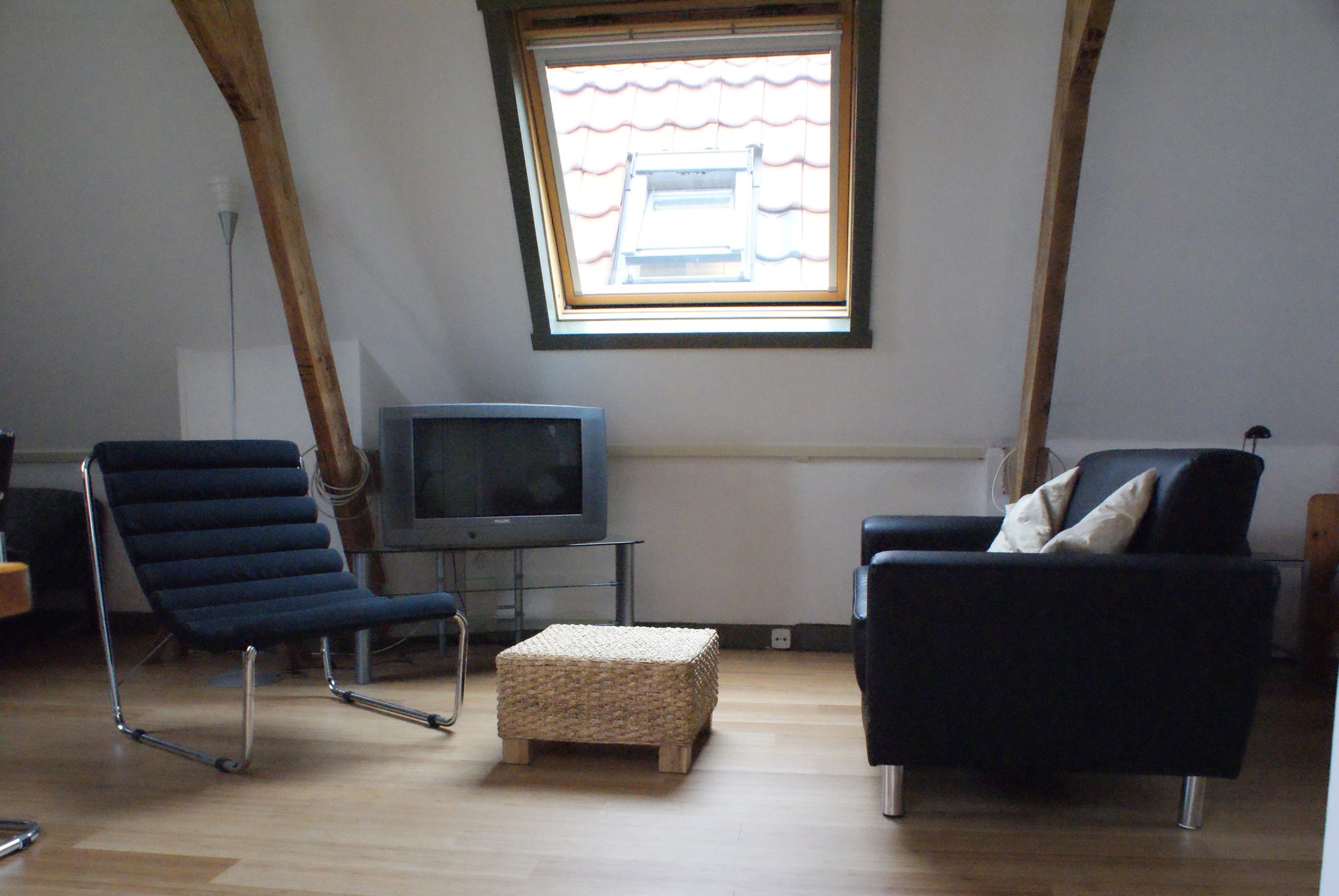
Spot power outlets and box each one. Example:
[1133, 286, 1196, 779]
[771, 629, 790, 648]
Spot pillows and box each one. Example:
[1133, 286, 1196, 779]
[987, 466, 1080, 552]
[1040, 467, 1156, 553]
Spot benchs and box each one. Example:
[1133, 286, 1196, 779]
[0, 488, 105, 613]
[851, 425, 1281, 831]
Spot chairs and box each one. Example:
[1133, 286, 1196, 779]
[82, 440, 467, 776]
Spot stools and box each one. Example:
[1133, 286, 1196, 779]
[495, 623, 718, 774]
[0, 561, 40, 858]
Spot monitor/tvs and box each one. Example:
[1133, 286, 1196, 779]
[378, 402, 609, 548]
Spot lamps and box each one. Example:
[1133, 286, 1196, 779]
[208, 173, 250, 440]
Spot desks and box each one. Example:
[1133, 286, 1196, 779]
[344, 534, 645, 685]
